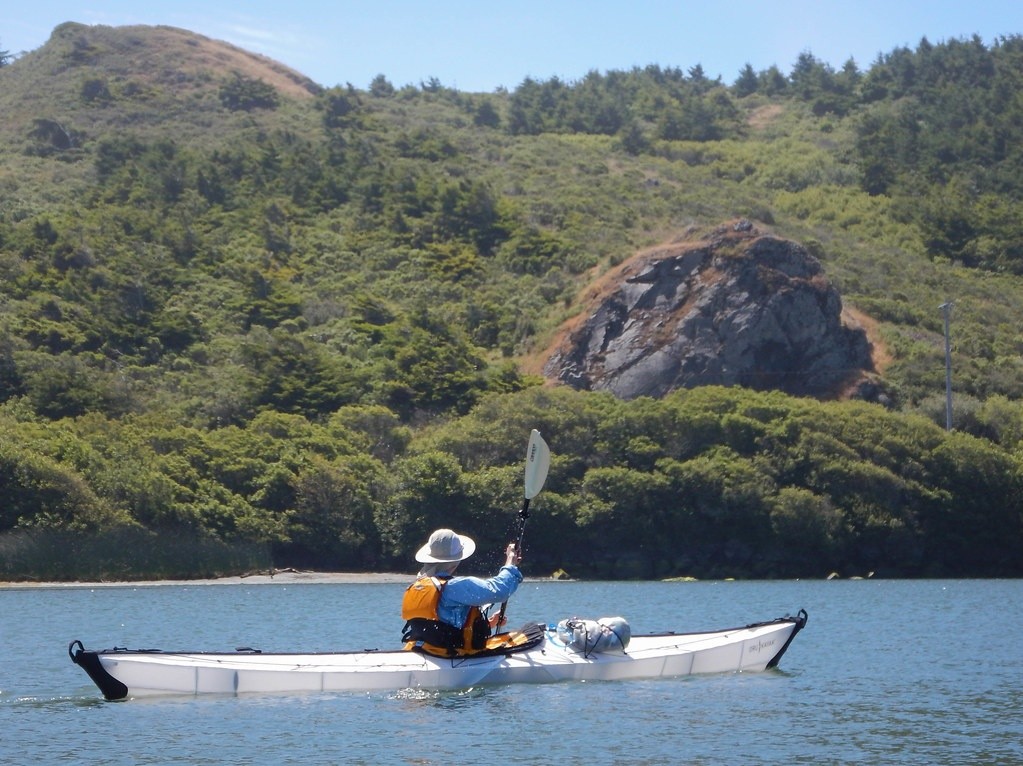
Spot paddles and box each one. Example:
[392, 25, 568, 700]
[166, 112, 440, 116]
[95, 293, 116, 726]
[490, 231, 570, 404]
[494, 427, 553, 638]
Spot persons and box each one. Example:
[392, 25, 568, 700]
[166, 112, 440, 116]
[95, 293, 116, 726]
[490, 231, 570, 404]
[402, 529, 523, 659]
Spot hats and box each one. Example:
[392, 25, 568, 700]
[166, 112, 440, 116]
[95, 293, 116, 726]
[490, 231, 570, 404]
[413, 528, 477, 563]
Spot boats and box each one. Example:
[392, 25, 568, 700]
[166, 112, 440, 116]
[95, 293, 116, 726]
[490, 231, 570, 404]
[66, 608, 807, 701]
